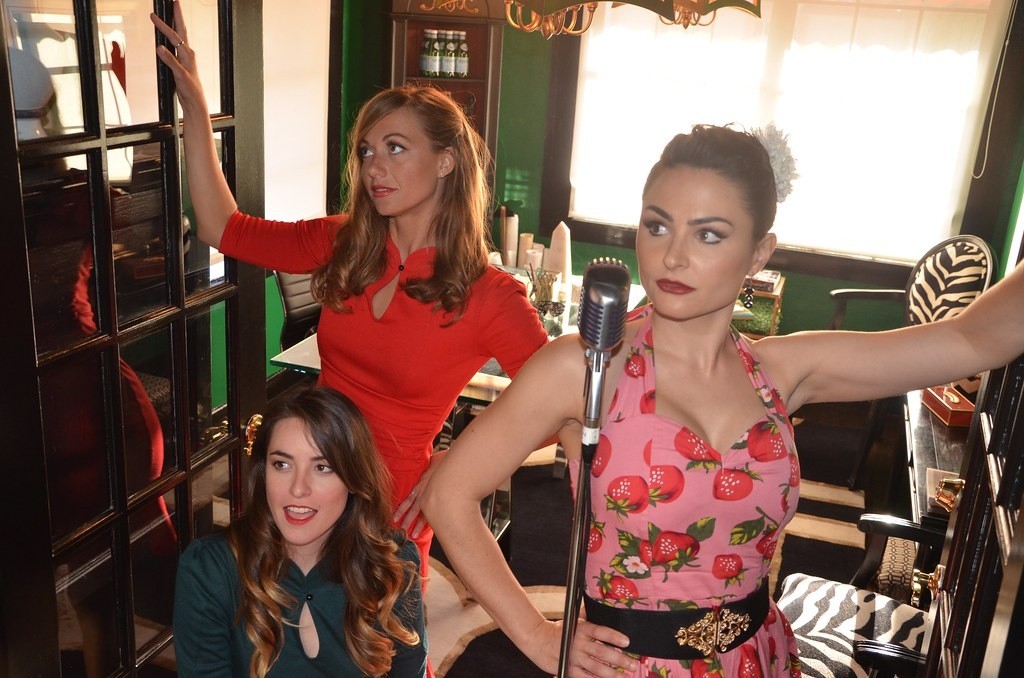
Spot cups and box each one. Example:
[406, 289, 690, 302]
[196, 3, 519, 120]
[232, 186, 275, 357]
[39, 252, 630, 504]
[518, 232, 550, 271]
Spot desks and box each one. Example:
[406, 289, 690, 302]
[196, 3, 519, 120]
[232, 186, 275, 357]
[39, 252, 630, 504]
[897, 382, 977, 530]
[269, 322, 527, 441]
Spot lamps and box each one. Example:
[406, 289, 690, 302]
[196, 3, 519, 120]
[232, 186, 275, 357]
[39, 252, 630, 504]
[418, 0, 762, 42]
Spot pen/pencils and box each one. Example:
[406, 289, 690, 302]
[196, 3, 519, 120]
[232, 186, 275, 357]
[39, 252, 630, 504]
[534, 267, 558, 302]
[524, 263, 536, 285]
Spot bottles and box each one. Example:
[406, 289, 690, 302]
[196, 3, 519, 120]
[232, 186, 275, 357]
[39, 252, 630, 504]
[500, 214, 519, 267]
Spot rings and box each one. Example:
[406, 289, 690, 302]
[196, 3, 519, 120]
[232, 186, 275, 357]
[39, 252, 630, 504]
[176, 41, 184, 48]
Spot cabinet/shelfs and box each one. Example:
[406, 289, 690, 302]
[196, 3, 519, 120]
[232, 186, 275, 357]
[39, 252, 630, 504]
[1, 0, 271, 677]
[926, 233, 1024, 678]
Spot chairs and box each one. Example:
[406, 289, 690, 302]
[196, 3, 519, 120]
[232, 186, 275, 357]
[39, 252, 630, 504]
[816, 233, 994, 491]
[772, 510, 949, 677]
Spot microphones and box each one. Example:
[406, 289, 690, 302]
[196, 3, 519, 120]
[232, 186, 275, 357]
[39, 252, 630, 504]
[577, 256, 632, 469]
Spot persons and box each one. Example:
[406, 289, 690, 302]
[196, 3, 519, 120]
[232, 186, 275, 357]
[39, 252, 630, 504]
[419, 125, 1024, 678]
[0, 44, 178, 678]
[171, 385, 428, 678]
[149, 0, 561, 678]
